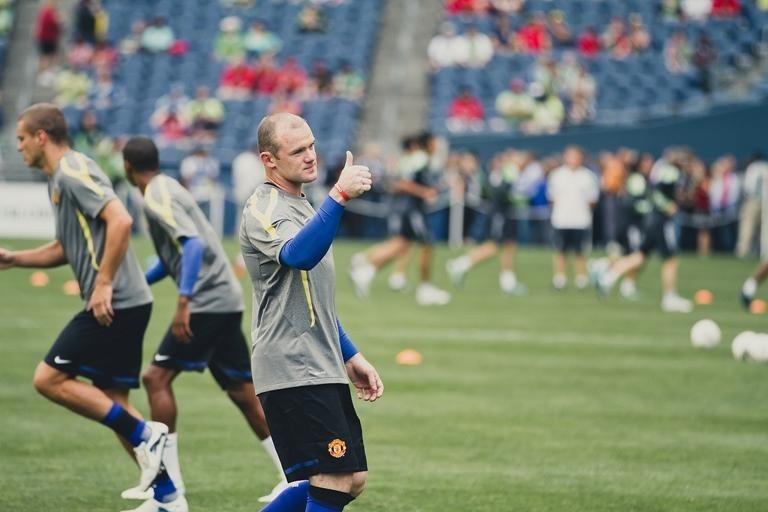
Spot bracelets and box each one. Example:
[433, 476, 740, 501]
[332, 183, 350, 202]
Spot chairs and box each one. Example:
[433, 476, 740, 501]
[0, 0, 767, 178]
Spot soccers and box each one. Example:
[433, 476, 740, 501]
[732, 329, 768, 364]
[690, 319, 721, 348]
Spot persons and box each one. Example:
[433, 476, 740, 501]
[0, 1, 366, 281]
[238, 111, 384, 512]
[115, 138, 310, 511]
[0, 104, 168, 500]
[354, 0, 767, 310]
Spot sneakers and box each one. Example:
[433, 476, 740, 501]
[133, 420, 169, 493]
[120, 480, 186, 500]
[346, 254, 526, 308]
[551, 257, 695, 314]
[256, 480, 309, 502]
[118, 495, 190, 512]
[739, 278, 760, 310]
